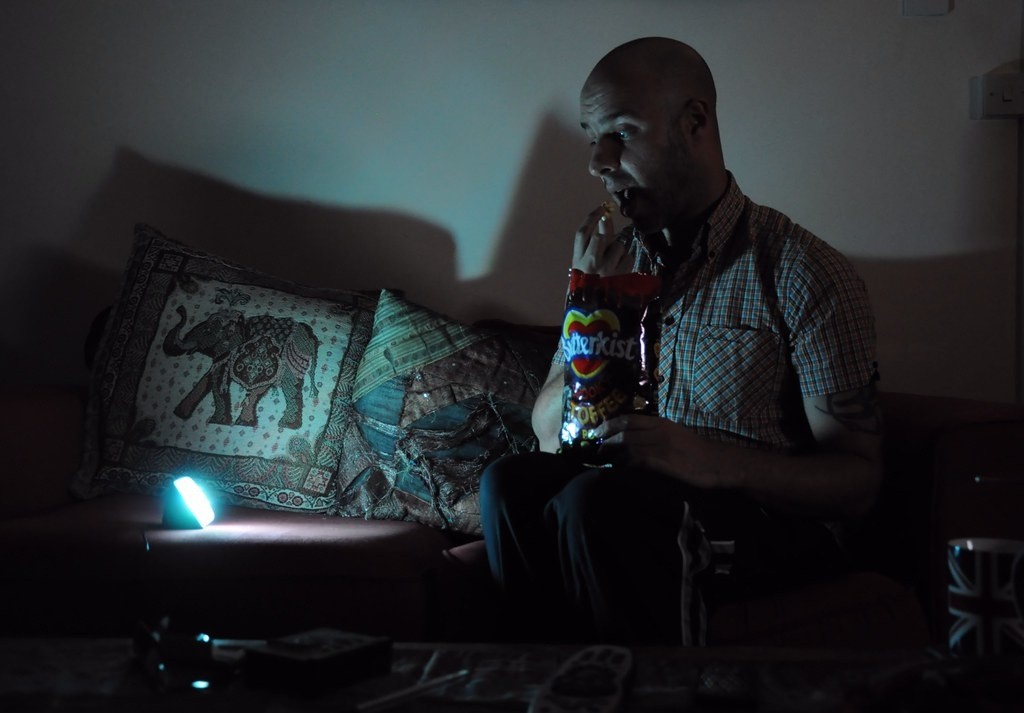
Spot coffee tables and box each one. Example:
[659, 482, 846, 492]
[0, 638, 1024, 713]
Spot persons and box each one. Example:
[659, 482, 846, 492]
[475, 32, 896, 663]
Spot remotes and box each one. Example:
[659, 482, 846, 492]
[527, 644, 632, 713]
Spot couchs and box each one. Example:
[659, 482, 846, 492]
[0, 318, 1024, 652]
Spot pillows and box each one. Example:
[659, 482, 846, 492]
[324, 287, 550, 538]
[71, 222, 406, 522]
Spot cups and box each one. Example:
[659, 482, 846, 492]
[947, 537, 1024, 620]
